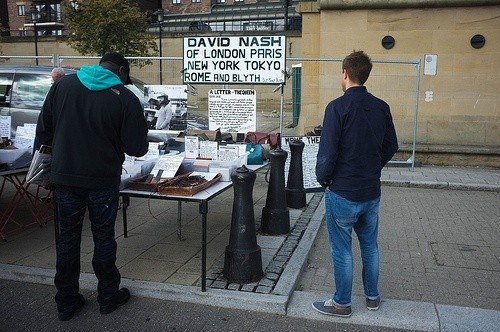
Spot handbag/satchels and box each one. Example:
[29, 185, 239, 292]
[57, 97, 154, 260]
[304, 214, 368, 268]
[244, 132, 281, 165]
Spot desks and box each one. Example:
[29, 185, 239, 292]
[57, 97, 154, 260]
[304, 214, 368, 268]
[118, 158, 271, 292]
[0, 166, 54, 243]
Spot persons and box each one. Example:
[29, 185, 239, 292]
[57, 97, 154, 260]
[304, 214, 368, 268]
[311, 49, 399, 317]
[33, 51, 150, 322]
[51, 67, 64, 82]
[154, 93, 172, 130]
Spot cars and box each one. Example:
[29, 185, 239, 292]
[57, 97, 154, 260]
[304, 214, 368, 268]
[0, 64, 188, 144]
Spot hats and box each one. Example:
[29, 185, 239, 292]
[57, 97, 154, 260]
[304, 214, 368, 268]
[99, 52, 132, 85]
[157, 93, 168, 98]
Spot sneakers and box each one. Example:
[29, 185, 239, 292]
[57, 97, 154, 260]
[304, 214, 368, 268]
[312, 299, 352, 318]
[58, 293, 86, 320]
[100, 288, 130, 314]
[366, 296, 382, 311]
